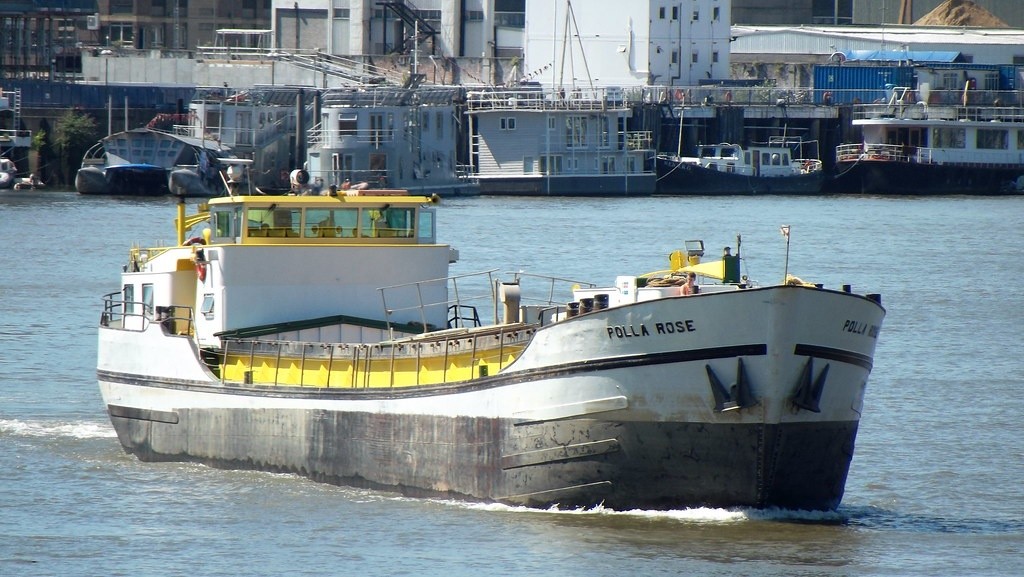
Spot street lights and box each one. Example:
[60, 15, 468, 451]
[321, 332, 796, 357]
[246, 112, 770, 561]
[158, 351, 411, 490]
[101, 50, 115, 138]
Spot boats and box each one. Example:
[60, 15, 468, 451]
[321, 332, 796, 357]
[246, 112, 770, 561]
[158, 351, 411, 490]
[76, 127, 251, 195]
[831, 82, 1024, 193]
[646, 94, 828, 194]
[258, 77, 479, 198]
[0, 159, 45, 191]
[457, 83, 658, 195]
[97, 149, 886, 513]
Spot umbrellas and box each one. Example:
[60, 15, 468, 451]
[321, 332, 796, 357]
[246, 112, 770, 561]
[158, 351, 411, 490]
[505, 63, 525, 106]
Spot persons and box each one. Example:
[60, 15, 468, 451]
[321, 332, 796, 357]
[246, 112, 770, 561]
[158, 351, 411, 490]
[704, 91, 714, 107]
[823, 91, 833, 107]
[724, 89, 734, 103]
[379, 175, 385, 190]
[680, 271, 696, 296]
[14, 168, 59, 190]
[342, 177, 351, 191]
[898, 140, 907, 162]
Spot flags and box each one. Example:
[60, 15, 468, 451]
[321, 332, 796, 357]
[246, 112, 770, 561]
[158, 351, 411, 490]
[781, 226, 789, 237]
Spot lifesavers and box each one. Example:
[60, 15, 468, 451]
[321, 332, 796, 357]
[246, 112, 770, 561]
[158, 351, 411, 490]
[674, 89, 683, 100]
[725, 92, 732, 102]
[804, 161, 811, 169]
[823, 91, 833, 102]
[182, 237, 206, 246]
[195, 262, 206, 281]
